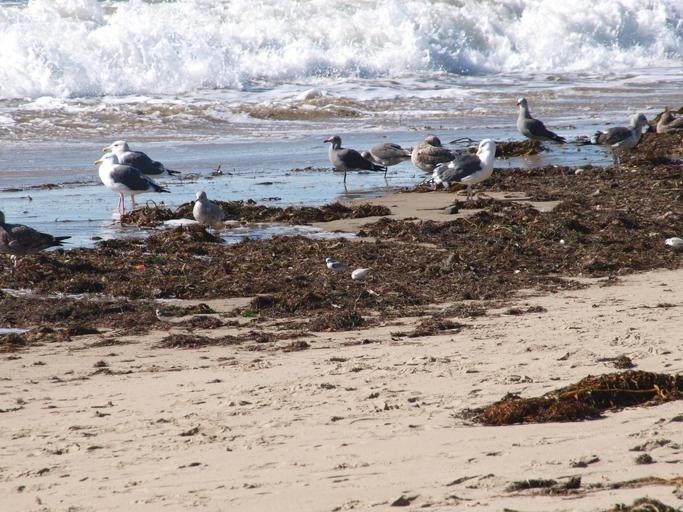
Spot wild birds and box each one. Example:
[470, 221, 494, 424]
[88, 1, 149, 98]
[517, 98, 567, 154]
[94, 152, 171, 216]
[192, 191, 226, 234]
[323, 134, 497, 203]
[325, 257, 349, 281]
[351, 266, 375, 281]
[103, 140, 182, 209]
[568, 112, 652, 165]
[664, 237, 683, 250]
[0, 211, 73, 269]
